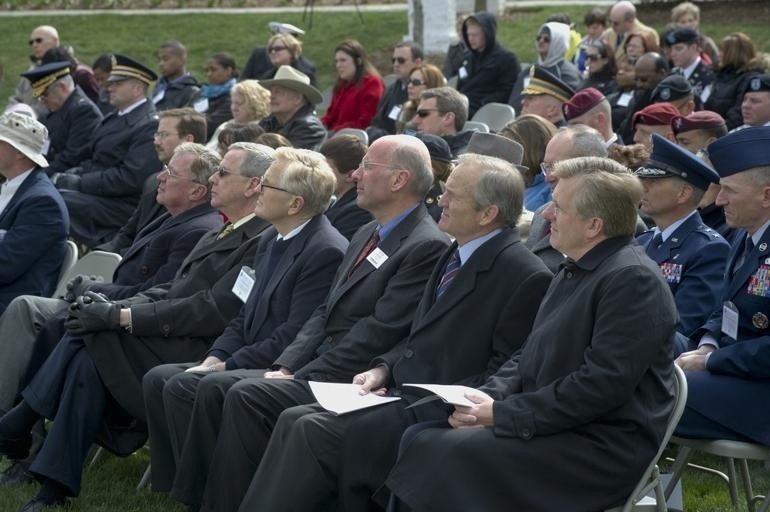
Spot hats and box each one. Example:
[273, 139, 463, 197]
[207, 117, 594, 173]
[457, 130, 530, 171]
[650, 75, 693, 102]
[521, 65, 575, 107]
[664, 26, 701, 47]
[20, 62, 72, 99]
[632, 103, 681, 131]
[744, 74, 770, 94]
[632, 133, 720, 192]
[562, 87, 606, 121]
[258, 65, 323, 106]
[671, 111, 727, 137]
[0, 113, 50, 168]
[708, 124, 770, 176]
[107, 54, 158, 86]
[412, 133, 453, 164]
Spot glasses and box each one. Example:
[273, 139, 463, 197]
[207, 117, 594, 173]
[358, 162, 396, 174]
[259, 176, 299, 197]
[536, 36, 549, 43]
[213, 166, 252, 178]
[407, 78, 421, 86]
[268, 47, 288, 53]
[415, 109, 447, 118]
[584, 54, 598, 61]
[28, 38, 42, 45]
[546, 200, 565, 217]
[161, 161, 201, 184]
[392, 56, 406, 64]
[153, 132, 187, 138]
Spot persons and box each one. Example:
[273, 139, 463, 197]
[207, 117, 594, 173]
[220, 124, 264, 155]
[660, 27, 714, 95]
[580, 9, 608, 51]
[383, 156, 678, 510]
[396, 63, 447, 133]
[267, 35, 315, 88]
[242, 21, 314, 83]
[414, 86, 477, 154]
[253, 133, 291, 152]
[456, 134, 534, 245]
[600, 0, 658, 61]
[520, 64, 574, 129]
[419, 134, 451, 223]
[457, 11, 517, 121]
[699, 32, 769, 128]
[671, 4, 718, 58]
[583, 41, 619, 97]
[207, 78, 269, 151]
[646, 74, 693, 120]
[259, 63, 328, 150]
[622, 54, 666, 110]
[321, 39, 384, 133]
[174, 135, 452, 511]
[633, 103, 677, 144]
[50, 54, 158, 258]
[20, 62, 102, 178]
[239, 154, 553, 510]
[1, 142, 276, 512]
[367, 41, 423, 140]
[563, 86, 622, 151]
[634, 133, 730, 355]
[9, 25, 60, 119]
[510, 23, 581, 115]
[143, 147, 349, 510]
[149, 40, 200, 112]
[2, 143, 223, 483]
[614, 33, 646, 86]
[41, 45, 100, 105]
[0, 110, 70, 314]
[669, 30, 720, 97]
[314, 134, 373, 241]
[742, 74, 770, 130]
[186, 51, 238, 142]
[524, 124, 650, 276]
[670, 111, 726, 151]
[672, 130, 769, 453]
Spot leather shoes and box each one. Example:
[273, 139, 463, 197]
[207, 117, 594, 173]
[19, 499, 73, 512]
[1, 463, 36, 488]
[0, 434, 33, 460]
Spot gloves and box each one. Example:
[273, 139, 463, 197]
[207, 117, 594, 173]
[66, 274, 104, 301]
[63, 291, 125, 334]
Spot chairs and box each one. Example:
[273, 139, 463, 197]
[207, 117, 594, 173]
[654, 425, 768, 510]
[607, 360, 687, 512]
[56, 250, 123, 303]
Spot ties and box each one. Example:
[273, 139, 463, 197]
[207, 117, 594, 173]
[646, 232, 663, 256]
[435, 247, 460, 301]
[216, 224, 233, 239]
[731, 236, 755, 273]
[348, 229, 380, 277]
